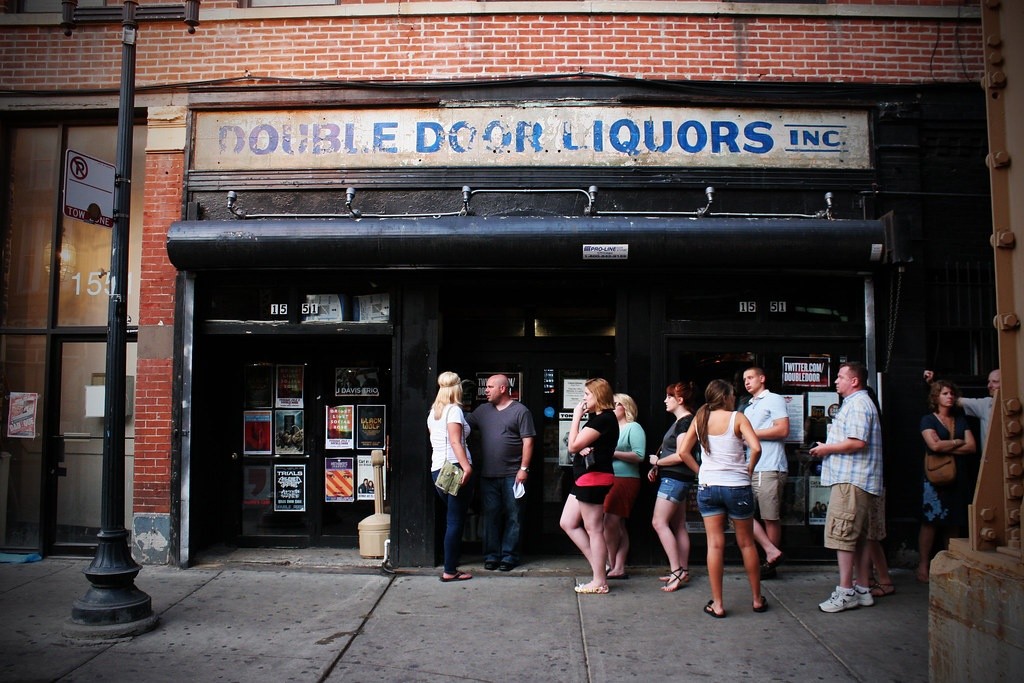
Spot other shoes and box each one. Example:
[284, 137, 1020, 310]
[484, 560, 499, 570]
[915, 569, 929, 590]
[500, 562, 514, 571]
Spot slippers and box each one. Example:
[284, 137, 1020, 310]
[440, 570, 472, 582]
[604, 567, 629, 579]
[753, 596, 769, 612]
[703, 600, 726, 619]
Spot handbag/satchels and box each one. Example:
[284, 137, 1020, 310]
[434, 459, 465, 497]
[923, 445, 958, 485]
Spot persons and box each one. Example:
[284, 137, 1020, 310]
[741, 367, 790, 580]
[647, 381, 699, 592]
[464, 375, 536, 569]
[923, 370, 1000, 457]
[426, 372, 474, 582]
[560, 377, 620, 594]
[918, 378, 977, 583]
[679, 380, 767, 618]
[601, 393, 646, 579]
[813, 362, 895, 613]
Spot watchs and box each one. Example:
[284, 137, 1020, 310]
[520, 466, 530, 473]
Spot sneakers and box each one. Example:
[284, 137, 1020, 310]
[818, 585, 859, 613]
[853, 584, 875, 606]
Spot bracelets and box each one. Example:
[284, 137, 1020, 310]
[953, 440, 959, 450]
[656, 458, 659, 466]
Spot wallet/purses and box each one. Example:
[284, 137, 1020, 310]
[584, 449, 596, 470]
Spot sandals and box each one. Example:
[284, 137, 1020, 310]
[852, 578, 897, 597]
[661, 567, 686, 592]
[659, 567, 689, 582]
[575, 581, 610, 593]
